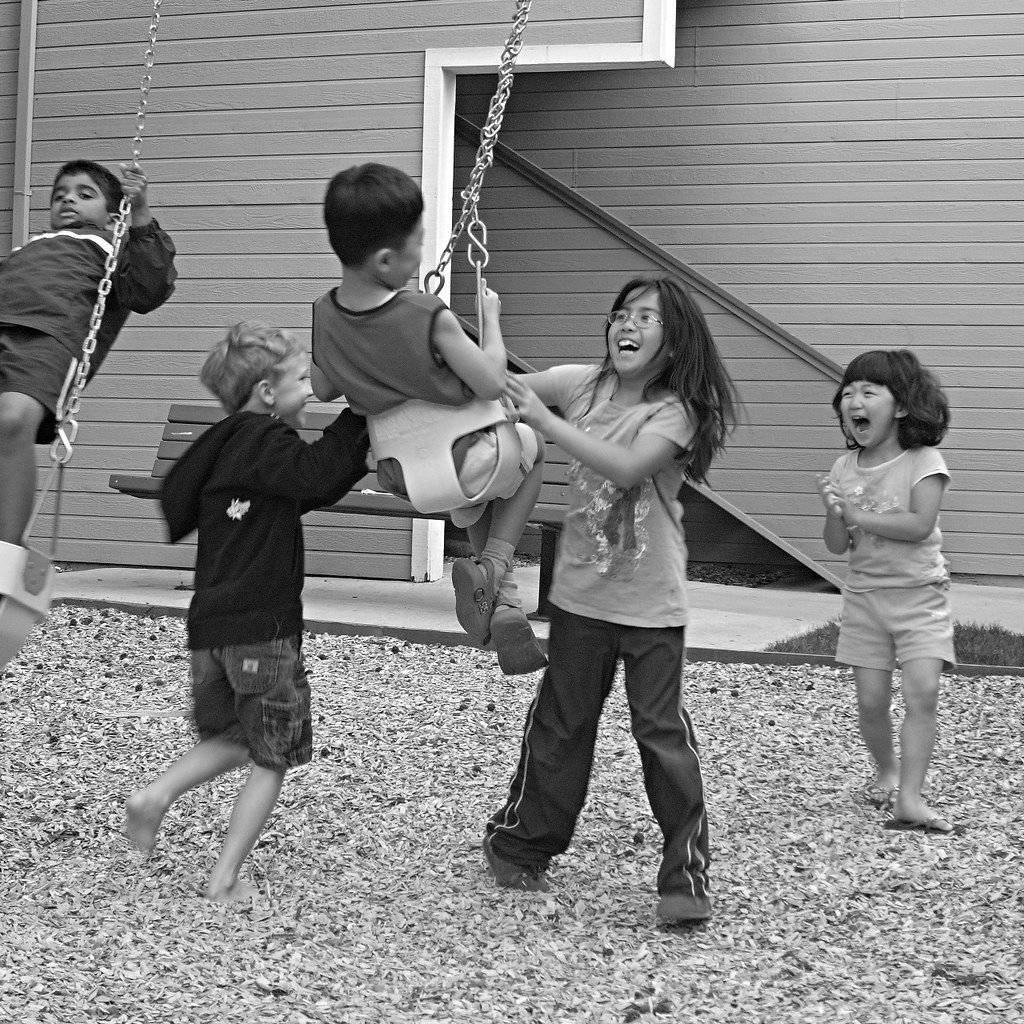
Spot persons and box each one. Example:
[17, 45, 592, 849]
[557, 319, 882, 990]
[307, 164, 546, 675]
[814, 349, 957, 834]
[122, 320, 371, 903]
[483, 275, 736, 918]
[0, 159, 177, 546]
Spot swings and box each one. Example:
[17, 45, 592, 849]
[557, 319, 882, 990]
[360, 2, 537, 514]
[0, 0, 164, 684]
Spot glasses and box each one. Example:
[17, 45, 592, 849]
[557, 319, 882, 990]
[607, 311, 663, 329]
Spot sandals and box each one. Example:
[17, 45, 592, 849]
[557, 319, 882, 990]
[451, 557, 499, 646]
[490, 605, 549, 677]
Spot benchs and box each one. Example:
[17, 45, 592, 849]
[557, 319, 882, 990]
[107, 402, 576, 624]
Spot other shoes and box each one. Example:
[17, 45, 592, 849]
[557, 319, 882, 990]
[658, 888, 712, 918]
[484, 833, 549, 893]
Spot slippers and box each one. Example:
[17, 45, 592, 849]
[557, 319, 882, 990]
[869, 784, 898, 806]
[885, 815, 965, 839]
